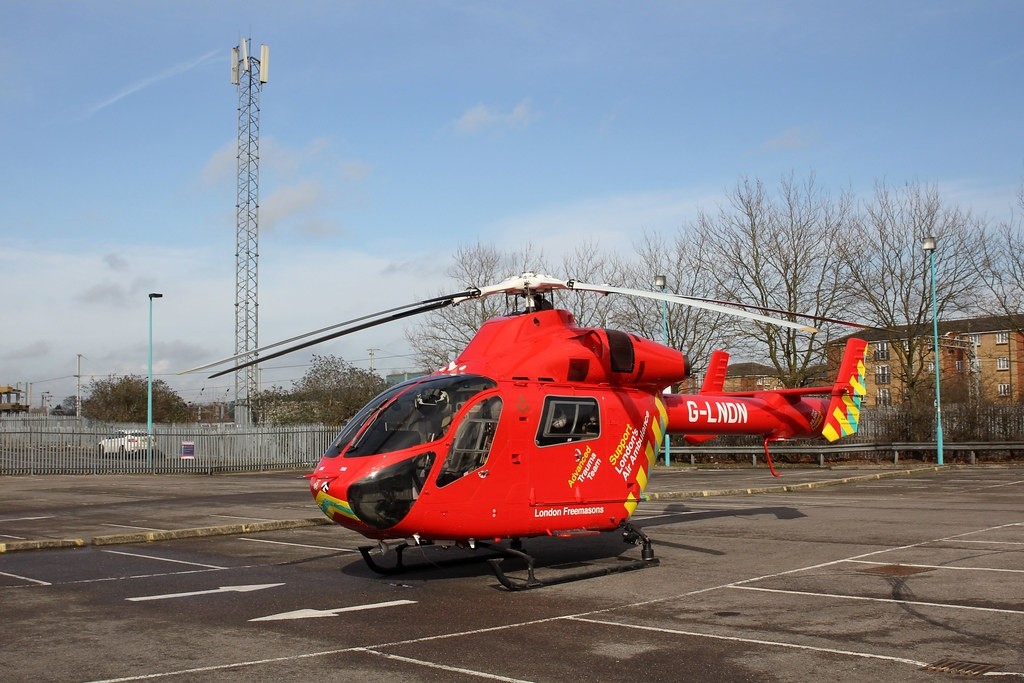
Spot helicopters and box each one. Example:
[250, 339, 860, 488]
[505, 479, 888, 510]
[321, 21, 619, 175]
[176, 270, 907, 593]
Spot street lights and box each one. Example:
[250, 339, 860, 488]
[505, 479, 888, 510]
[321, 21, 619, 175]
[653, 274, 672, 469]
[921, 237, 945, 465]
[147, 292, 164, 475]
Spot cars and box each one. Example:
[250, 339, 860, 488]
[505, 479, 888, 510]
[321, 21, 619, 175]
[97, 429, 158, 461]
